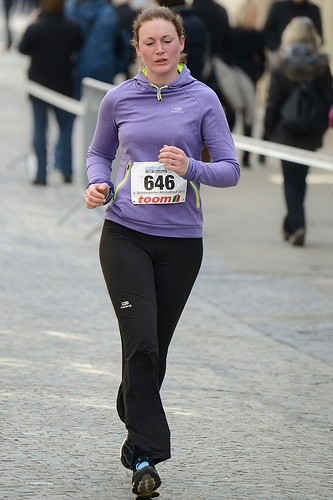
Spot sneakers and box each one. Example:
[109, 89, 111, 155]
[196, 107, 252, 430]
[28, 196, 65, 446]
[132, 457, 161, 500]
[120, 435, 134, 470]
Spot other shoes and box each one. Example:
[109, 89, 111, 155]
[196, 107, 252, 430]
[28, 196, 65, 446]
[283, 217, 305, 246]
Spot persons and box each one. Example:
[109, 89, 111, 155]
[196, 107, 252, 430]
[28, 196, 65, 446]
[84, 6, 240, 496]
[18, 0, 333, 246]
